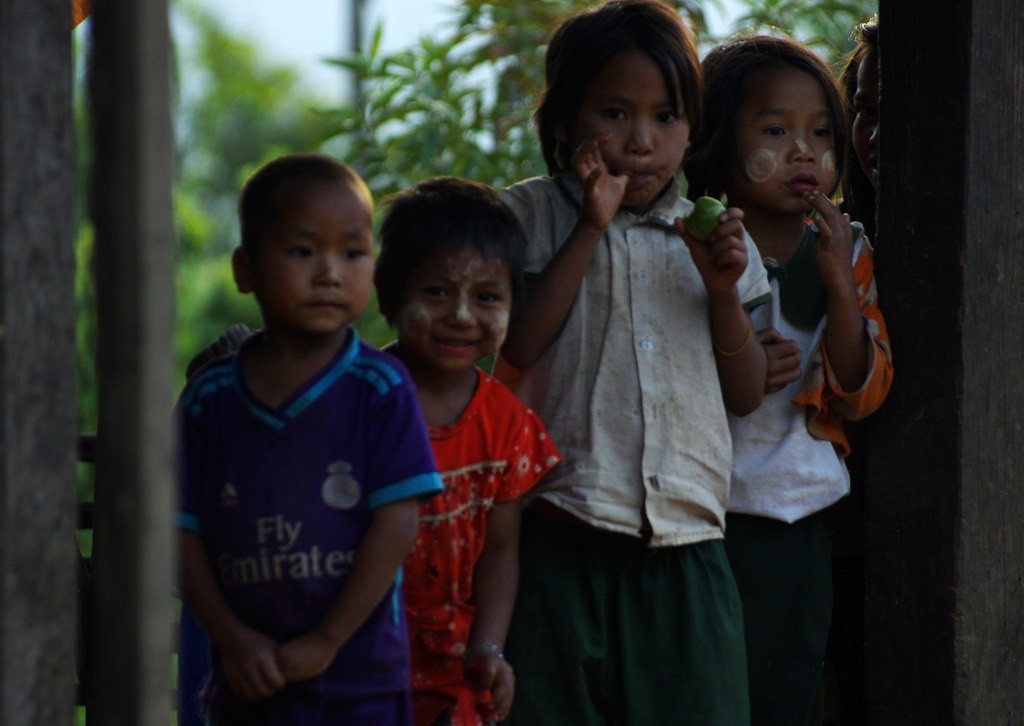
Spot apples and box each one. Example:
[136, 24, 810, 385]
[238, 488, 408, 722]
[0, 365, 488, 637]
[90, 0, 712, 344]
[683, 195, 727, 240]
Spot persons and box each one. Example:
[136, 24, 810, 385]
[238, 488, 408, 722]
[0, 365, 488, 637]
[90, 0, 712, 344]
[377, 176, 565, 726]
[837, 15, 879, 256]
[485, 0, 773, 725]
[165, 155, 445, 726]
[681, 33, 894, 725]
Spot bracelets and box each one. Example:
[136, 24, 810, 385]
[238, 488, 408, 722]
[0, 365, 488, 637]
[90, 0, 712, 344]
[715, 324, 752, 357]
